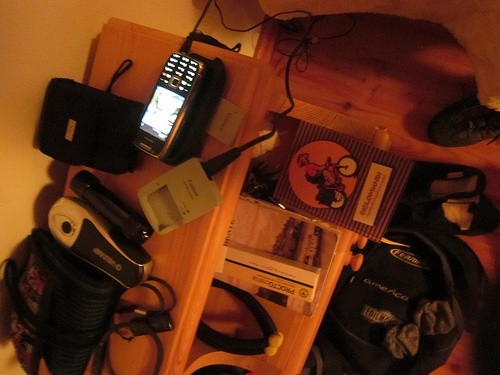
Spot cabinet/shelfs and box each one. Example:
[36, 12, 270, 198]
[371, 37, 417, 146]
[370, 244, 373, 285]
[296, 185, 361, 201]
[62, 18, 393, 374]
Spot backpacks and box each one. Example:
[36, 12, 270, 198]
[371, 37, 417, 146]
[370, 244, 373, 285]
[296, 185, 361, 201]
[320, 225, 492, 374]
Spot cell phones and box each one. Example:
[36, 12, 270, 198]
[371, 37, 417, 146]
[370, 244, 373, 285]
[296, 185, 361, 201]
[131, 51, 206, 162]
